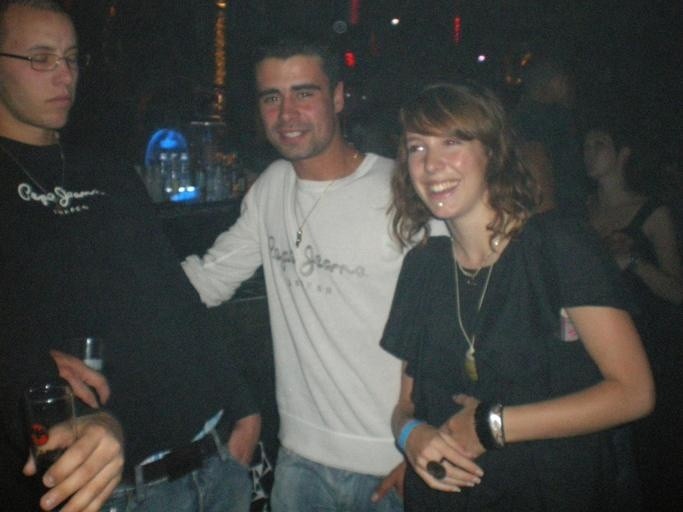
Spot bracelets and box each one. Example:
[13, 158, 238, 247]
[488, 404, 506, 446]
[475, 401, 501, 449]
[397, 419, 424, 452]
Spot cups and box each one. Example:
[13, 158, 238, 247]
[64, 335, 106, 411]
[22, 379, 83, 512]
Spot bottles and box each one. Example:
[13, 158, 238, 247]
[131, 137, 248, 211]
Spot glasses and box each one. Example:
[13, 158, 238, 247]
[0, 51, 92, 71]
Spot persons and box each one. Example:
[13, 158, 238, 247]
[378, 76, 658, 511]
[180, 30, 451, 512]
[511, 53, 682, 512]
[1, 1, 263, 512]
[2, 409, 126, 512]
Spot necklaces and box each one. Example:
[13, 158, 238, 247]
[456, 245, 499, 285]
[1, 136, 66, 215]
[452, 234, 500, 383]
[292, 148, 358, 246]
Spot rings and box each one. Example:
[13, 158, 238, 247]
[428, 455, 446, 479]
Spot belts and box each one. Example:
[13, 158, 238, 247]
[123, 409, 236, 487]
